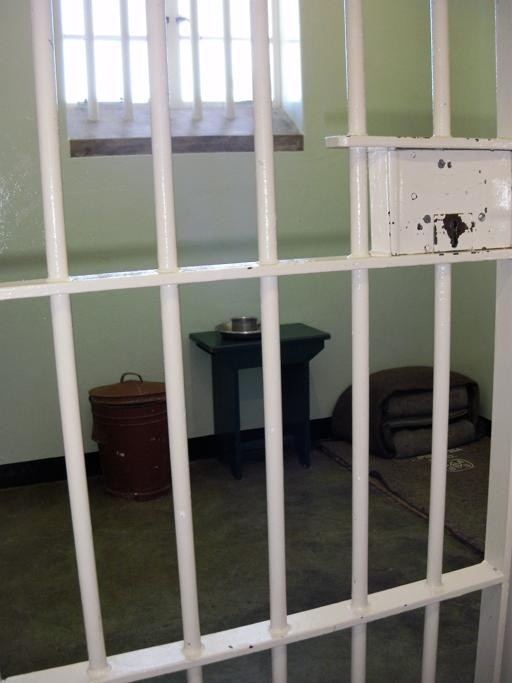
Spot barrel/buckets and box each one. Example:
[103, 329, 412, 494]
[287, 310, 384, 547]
[87, 371, 169, 500]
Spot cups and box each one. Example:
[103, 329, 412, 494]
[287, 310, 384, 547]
[230, 316, 258, 331]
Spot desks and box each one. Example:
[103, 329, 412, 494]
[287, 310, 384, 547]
[189, 322, 332, 481]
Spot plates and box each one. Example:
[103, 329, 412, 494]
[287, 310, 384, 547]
[213, 321, 261, 341]
